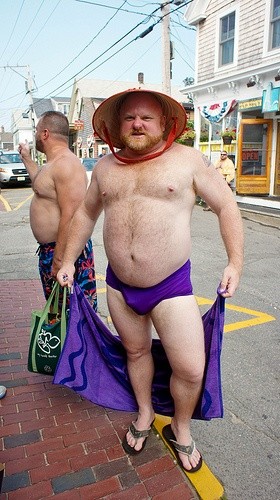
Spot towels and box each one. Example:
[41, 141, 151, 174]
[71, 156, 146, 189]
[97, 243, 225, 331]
[52, 280, 225, 421]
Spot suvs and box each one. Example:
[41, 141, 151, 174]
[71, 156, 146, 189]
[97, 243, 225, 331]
[0, 150, 32, 186]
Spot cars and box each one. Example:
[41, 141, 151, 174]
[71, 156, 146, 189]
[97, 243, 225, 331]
[79, 158, 99, 189]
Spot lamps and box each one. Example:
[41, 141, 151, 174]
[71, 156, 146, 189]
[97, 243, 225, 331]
[247, 75, 260, 87]
[275, 69, 280, 81]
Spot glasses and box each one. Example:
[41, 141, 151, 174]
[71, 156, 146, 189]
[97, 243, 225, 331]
[221, 154, 227, 156]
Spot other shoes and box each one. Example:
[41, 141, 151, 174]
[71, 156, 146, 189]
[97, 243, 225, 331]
[203, 207, 212, 211]
[0, 386, 6, 399]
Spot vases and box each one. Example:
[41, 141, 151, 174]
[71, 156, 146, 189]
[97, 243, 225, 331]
[223, 137, 232, 144]
[184, 139, 193, 147]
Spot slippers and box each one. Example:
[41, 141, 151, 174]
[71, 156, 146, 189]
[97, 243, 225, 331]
[162, 424, 202, 473]
[122, 418, 155, 455]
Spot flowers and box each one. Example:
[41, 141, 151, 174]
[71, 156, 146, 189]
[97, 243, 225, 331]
[216, 128, 237, 140]
[176, 126, 196, 143]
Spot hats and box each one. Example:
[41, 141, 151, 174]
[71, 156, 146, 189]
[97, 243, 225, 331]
[92, 88, 187, 150]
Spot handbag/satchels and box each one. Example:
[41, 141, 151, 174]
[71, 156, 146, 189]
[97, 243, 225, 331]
[27, 281, 69, 376]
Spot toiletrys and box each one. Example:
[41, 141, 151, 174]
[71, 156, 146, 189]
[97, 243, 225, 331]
[50, 317, 56, 324]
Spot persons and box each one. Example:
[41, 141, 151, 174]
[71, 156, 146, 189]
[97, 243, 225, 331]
[55, 85, 245, 473]
[18, 111, 96, 324]
[204, 150, 235, 213]
